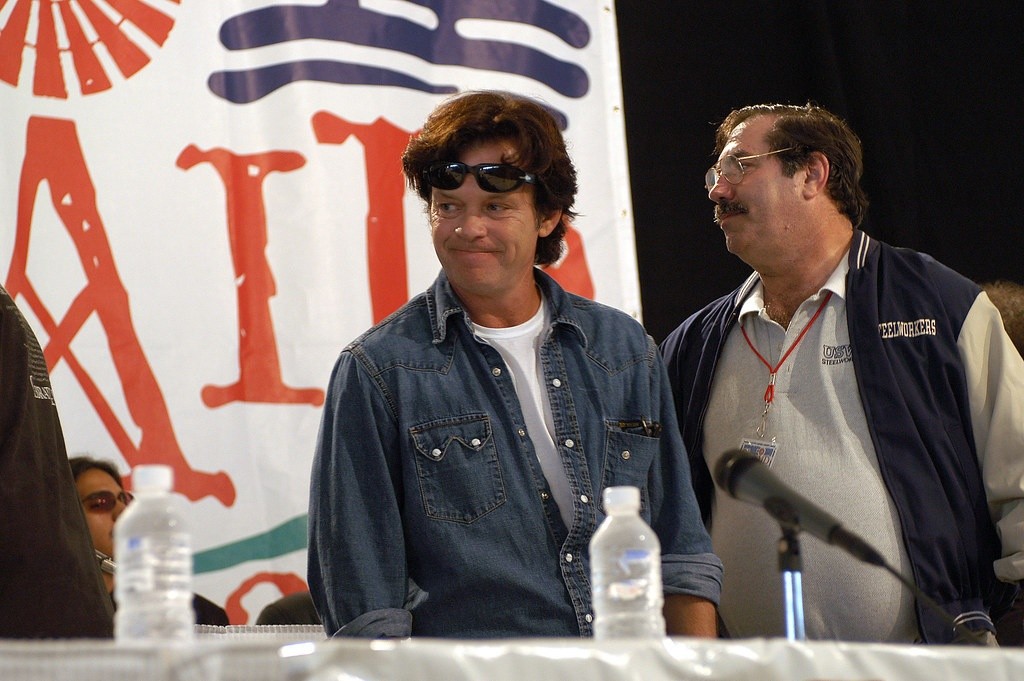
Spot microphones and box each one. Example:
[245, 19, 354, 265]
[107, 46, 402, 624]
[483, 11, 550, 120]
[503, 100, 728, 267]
[714, 448, 886, 565]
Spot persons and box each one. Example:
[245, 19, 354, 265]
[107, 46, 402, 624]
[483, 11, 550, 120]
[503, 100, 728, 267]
[306, 90, 726, 640]
[0, 287, 323, 640]
[657, 103, 1024, 642]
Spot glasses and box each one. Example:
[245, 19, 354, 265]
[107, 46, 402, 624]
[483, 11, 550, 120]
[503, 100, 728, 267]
[422, 159, 537, 194]
[79, 491, 134, 515]
[705, 146, 809, 192]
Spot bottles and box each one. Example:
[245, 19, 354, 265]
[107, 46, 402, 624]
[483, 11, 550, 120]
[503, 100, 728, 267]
[590, 485, 664, 642]
[113, 465, 193, 642]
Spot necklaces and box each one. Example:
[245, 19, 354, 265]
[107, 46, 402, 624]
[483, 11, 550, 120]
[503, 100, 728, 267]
[768, 313, 793, 327]
